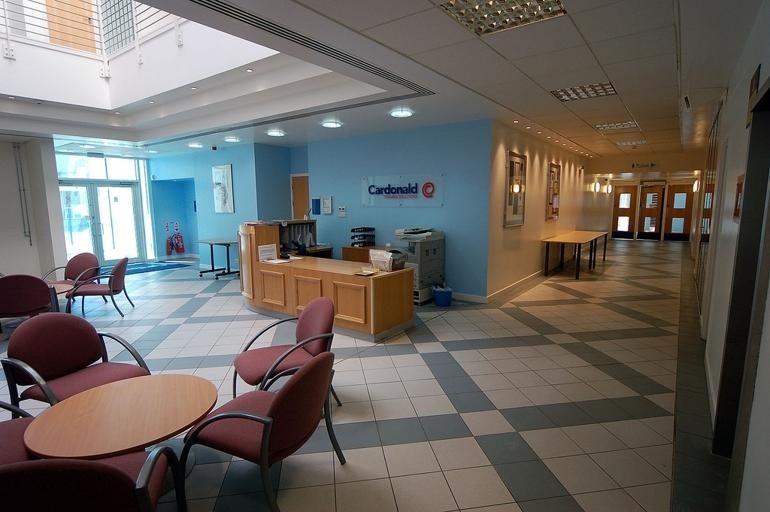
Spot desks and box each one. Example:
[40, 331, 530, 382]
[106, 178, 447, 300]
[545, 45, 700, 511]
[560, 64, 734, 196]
[192, 240, 239, 280]
[46, 283, 75, 296]
[24, 373, 218, 496]
[180, 350, 346, 511]
[341, 246, 387, 263]
[232, 296, 343, 418]
[542, 231, 609, 280]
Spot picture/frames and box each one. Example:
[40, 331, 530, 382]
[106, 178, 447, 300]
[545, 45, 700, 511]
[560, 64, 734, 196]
[321, 195, 331, 214]
[733, 173, 745, 219]
[546, 162, 560, 222]
[503, 149, 526, 228]
[212, 164, 234, 213]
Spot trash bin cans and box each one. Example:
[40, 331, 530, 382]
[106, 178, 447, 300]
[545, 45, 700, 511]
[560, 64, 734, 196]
[432, 287, 453, 306]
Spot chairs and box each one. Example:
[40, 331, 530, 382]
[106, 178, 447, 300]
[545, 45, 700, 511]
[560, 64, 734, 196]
[41, 253, 108, 316]
[1, 416, 180, 511]
[64, 256, 135, 318]
[1, 274, 60, 319]
[1, 311, 151, 419]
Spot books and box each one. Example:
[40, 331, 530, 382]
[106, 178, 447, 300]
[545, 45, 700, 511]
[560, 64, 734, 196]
[280, 224, 312, 249]
[368, 248, 393, 272]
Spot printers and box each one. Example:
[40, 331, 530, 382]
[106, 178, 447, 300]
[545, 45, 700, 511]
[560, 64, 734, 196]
[393, 227, 445, 307]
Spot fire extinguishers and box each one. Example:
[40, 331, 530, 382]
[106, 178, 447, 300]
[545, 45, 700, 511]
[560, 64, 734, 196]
[167, 230, 184, 256]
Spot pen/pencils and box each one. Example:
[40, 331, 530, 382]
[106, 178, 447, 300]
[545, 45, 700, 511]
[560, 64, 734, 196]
[308, 208, 311, 215]
